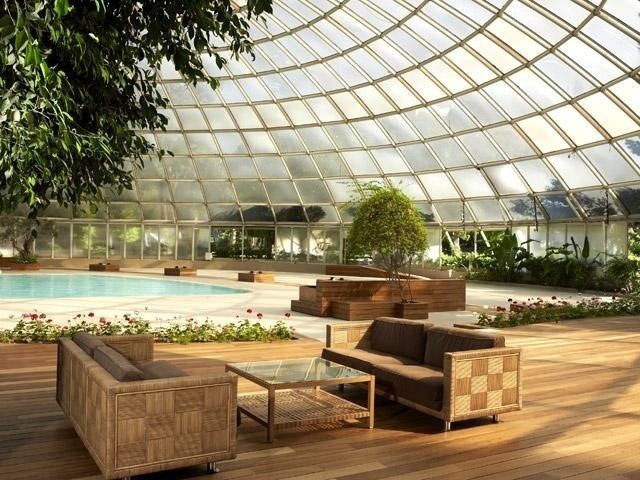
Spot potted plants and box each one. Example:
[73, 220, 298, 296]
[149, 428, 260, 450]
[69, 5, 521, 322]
[374, 209, 434, 320]
[8, 252, 40, 269]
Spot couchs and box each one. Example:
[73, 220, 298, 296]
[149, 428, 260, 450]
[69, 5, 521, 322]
[54, 332, 242, 479]
[321, 313, 523, 432]
